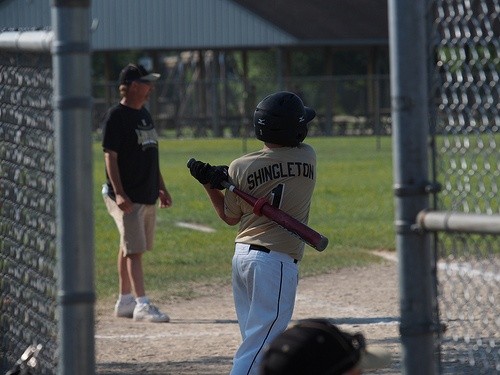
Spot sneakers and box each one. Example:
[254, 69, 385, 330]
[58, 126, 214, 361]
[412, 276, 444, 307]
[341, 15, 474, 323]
[133, 304, 169, 322]
[114, 300, 137, 317]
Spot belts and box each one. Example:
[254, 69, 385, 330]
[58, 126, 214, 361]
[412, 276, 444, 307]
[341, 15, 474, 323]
[249, 244, 298, 264]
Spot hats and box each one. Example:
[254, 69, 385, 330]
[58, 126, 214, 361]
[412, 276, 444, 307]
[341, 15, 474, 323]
[119, 64, 161, 85]
[261, 320, 360, 375]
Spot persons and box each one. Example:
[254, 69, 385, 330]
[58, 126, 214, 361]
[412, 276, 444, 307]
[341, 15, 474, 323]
[259, 318, 392, 375]
[191, 92, 317, 375]
[102, 64, 172, 322]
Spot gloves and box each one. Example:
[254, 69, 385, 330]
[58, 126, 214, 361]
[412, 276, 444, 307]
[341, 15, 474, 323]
[190, 161, 213, 185]
[210, 165, 229, 190]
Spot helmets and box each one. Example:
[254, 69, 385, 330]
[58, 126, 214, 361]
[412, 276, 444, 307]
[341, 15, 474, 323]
[253, 91, 316, 146]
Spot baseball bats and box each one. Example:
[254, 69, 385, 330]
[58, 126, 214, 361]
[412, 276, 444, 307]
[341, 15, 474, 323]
[186, 157, 329, 253]
